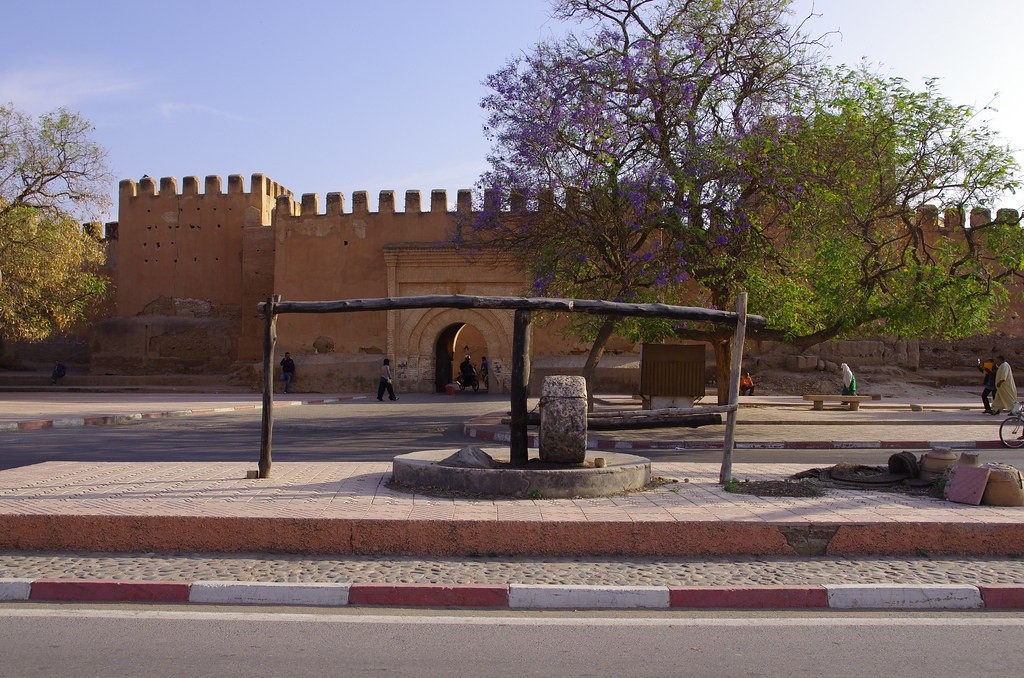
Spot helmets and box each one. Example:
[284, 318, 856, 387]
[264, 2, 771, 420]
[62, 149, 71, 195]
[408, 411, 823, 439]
[465, 355, 470, 360]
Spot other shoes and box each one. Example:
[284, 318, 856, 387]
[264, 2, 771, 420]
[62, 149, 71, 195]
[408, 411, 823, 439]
[283, 391, 286, 394]
[1008, 412, 1014, 416]
[749, 393, 754, 396]
[982, 409, 991, 413]
[988, 410, 995, 415]
[997, 409, 1000, 414]
[841, 402, 848, 405]
[742, 393, 745, 396]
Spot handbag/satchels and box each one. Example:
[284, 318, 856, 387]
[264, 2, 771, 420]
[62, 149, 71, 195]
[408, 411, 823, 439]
[387, 379, 393, 384]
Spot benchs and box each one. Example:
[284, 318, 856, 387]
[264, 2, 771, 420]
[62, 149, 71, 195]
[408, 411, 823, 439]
[802, 394, 872, 412]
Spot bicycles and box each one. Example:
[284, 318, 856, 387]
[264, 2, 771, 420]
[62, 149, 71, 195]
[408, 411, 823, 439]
[482, 374, 488, 389]
[999, 401, 1024, 449]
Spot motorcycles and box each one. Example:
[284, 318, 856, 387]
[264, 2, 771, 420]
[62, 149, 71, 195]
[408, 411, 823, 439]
[454, 373, 480, 393]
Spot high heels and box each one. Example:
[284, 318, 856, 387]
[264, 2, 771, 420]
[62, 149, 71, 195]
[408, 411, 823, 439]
[390, 397, 399, 401]
[377, 397, 383, 401]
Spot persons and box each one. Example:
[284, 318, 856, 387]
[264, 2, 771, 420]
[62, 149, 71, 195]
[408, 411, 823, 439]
[841, 363, 856, 405]
[481, 356, 488, 382]
[377, 359, 398, 401]
[280, 352, 295, 394]
[460, 355, 477, 387]
[51, 361, 67, 384]
[977, 359, 1000, 414]
[741, 372, 755, 396]
[988, 355, 1017, 416]
